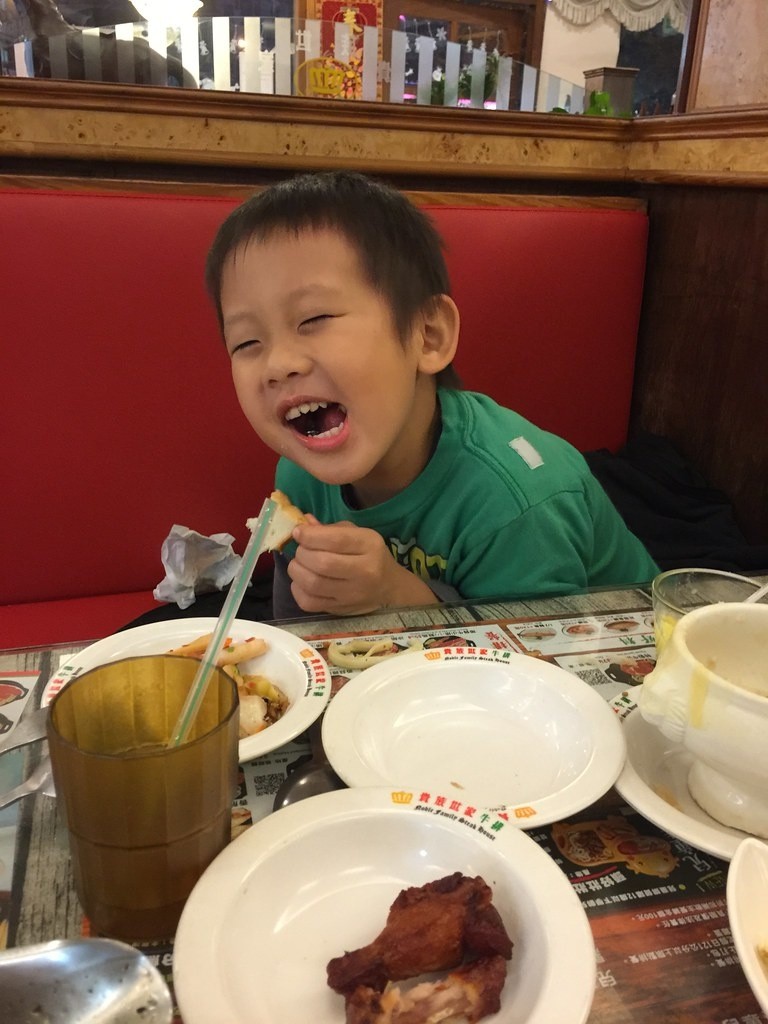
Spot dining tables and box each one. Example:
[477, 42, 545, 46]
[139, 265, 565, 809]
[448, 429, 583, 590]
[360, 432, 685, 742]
[0, 575, 768, 1024]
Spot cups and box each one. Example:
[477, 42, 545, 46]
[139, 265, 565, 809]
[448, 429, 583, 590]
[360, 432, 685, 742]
[650, 567, 768, 663]
[45, 654, 240, 958]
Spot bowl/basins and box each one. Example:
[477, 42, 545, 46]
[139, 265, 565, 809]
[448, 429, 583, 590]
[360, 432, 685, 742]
[635, 602, 768, 841]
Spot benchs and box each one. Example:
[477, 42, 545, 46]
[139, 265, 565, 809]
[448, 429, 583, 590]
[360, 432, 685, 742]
[0, 175, 649, 653]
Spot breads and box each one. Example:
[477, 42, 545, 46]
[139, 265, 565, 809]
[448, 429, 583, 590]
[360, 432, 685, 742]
[247, 490, 311, 552]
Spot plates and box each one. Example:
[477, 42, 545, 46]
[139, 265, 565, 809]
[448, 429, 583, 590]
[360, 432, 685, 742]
[321, 646, 628, 832]
[608, 684, 768, 862]
[725, 836, 768, 1021]
[40, 617, 334, 765]
[171, 785, 598, 1024]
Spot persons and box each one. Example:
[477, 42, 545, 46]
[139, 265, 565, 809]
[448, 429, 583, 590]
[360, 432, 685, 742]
[115, 171, 663, 633]
